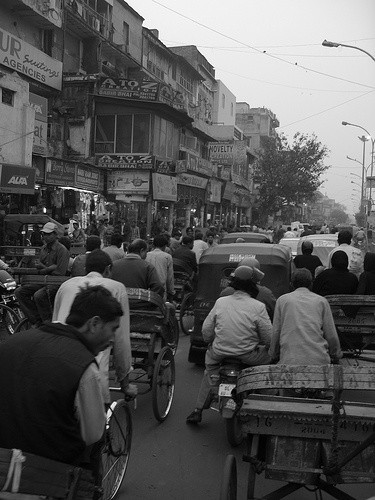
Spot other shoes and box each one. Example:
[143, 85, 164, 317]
[186, 410, 202, 425]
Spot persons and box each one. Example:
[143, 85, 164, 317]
[0, 219, 375, 469]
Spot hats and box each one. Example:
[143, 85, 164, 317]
[39, 222, 58, 236]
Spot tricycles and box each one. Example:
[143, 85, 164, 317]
[0, 214, 375, 500]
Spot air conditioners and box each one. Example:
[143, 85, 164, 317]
[72, 0, 82, 17]
[89, 16, 100, 32]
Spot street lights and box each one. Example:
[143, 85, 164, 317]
[321, 38, 375, 212]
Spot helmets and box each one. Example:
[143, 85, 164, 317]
[237, 257, 261, 269]
[230, 266, 264, 281]
